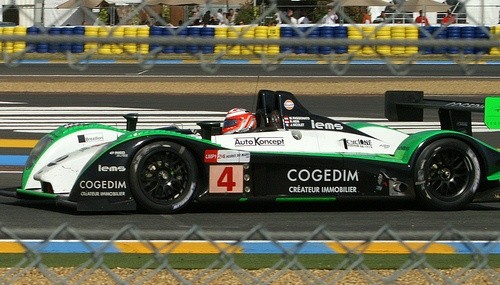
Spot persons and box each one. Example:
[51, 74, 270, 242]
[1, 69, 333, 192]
[81, 9, 456, 25]
[222, 108, 258, 134]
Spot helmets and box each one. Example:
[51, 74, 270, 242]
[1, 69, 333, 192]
[222, 108, 256, 134]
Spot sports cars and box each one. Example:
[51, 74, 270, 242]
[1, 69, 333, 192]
[14, 87, 500, 211]
